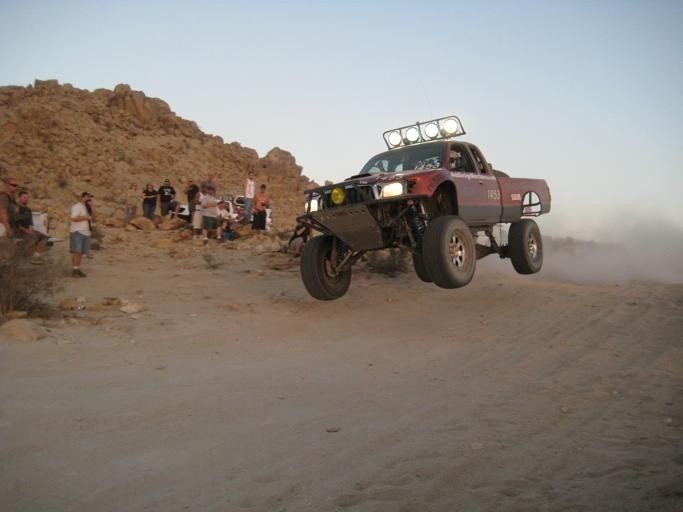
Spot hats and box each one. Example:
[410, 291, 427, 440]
[81, 191, 93, 196]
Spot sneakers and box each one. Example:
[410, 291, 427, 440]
[31, 257, 42, 264]
[203, 238, 208, 245]
[217, 238, 223, 242]
[71, 269, 86, 278]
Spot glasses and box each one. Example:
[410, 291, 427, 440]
[11, 182, 18, 187]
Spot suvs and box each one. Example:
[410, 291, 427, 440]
[176, 192, 273, 231]
[288, 115, 552, 302]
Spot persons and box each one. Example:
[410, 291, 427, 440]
[125, 171, 271, 245]
[80, 198, 94, 259]
[67, 191, 93, 278]
[287, 215, 312, 258]
[16, 190, 49, 265]
[0, 178, 19, 238]
[449, 150, 464, 171]
